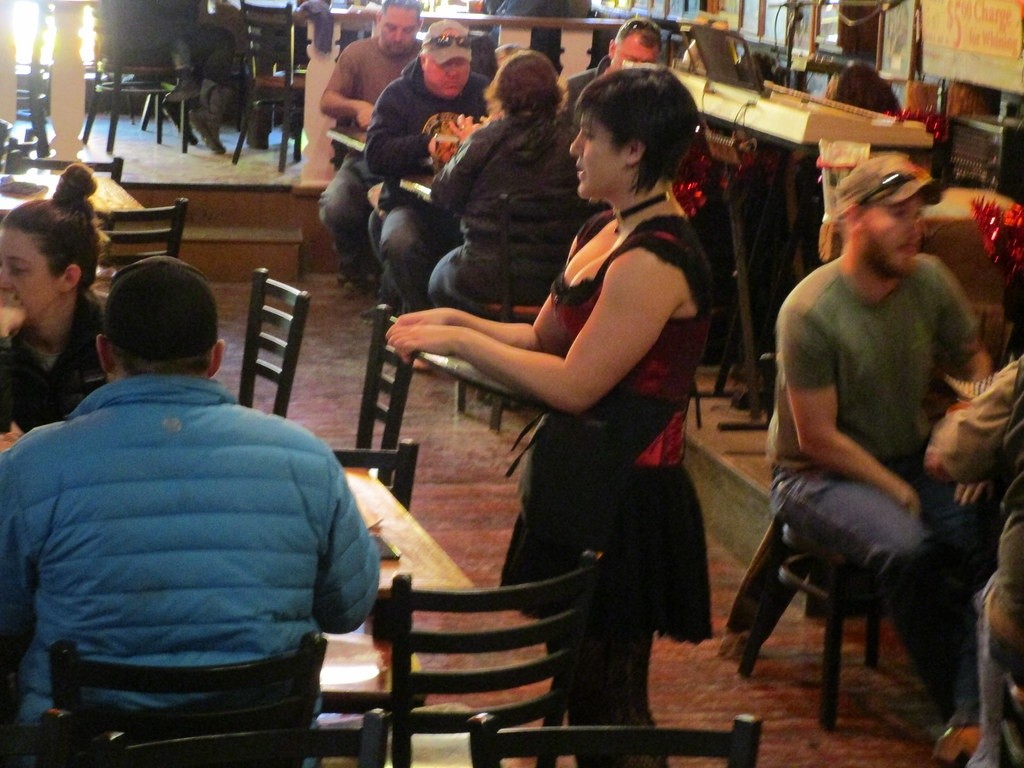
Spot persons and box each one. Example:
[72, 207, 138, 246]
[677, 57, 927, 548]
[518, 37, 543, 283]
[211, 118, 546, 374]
[928, 353, 1024, 762]
[384, 69, 713, 768]
[363, 19, 490, 306]
[99, 0, 236, 155]
[566, 17, 663, 108]
[0, 163, 107, 452]
[766, 154, 1004, 714]
[427, 49, 597, 309]
[0, 256, 380, 768]
[318, 0, 424, 284]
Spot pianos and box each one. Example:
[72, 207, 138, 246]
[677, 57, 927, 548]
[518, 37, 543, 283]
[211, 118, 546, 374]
[625, 58, 939, 438]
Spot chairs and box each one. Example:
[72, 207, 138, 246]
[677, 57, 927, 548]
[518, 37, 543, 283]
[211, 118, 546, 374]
[0, 0, 1024, 768]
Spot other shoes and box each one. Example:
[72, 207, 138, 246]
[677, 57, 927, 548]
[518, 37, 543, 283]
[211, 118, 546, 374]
[188, 106, 226, 154]
[161, 96, 197, 146]
[339, 270, 371, 287]
[385, 345, 430, 373]
[360, 299, 400, 325]
[929, 725, 981, 763]
[475, 387, 521, 411]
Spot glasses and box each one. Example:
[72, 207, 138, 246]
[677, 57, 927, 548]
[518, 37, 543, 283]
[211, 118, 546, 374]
[618, 20, 664, 39]
[855, 172, 917, 206]
[421, 33, 470, 49]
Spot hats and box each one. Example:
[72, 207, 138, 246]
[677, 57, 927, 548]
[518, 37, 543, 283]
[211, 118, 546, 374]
[103, 255, 219, 361]
[833, 150, 943, 217]
[422, 19, 472, 65]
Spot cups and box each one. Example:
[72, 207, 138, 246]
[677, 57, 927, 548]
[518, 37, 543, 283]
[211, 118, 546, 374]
[433, 136, 461, 181]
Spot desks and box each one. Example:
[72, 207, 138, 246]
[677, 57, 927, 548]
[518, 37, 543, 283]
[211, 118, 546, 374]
[398, 175, 433, 203]
[328, 125, 367, 153]
[0, 175, 147, 256]
[343, 464, 474, 639]
